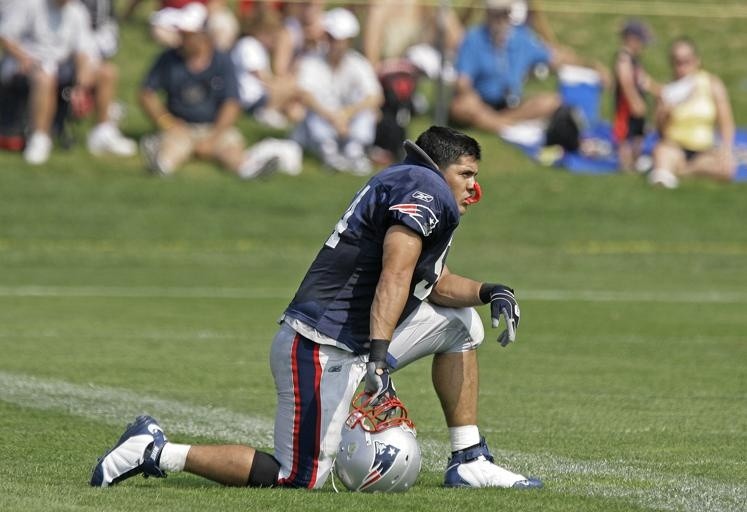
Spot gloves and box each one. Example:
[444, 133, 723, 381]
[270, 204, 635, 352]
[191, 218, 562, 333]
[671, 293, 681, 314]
[489, 284, 521, 348]
[364, 361, 400, 418]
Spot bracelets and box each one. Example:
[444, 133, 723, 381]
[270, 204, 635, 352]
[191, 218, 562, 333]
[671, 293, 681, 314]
[480, 279, 498, 302]
[369, 340, 388, 359]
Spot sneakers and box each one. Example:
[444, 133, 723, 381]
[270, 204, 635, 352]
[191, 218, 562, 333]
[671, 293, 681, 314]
[90, 416, 166, 488]
[445, 446, 545, 490]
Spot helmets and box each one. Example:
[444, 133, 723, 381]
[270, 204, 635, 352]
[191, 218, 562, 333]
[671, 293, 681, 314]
[331, 393, 423, 494]
[406, 42, 443, 80]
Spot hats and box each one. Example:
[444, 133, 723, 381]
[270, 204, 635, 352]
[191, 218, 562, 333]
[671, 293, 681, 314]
[172, 1, 207, 34]
[319, 5, 359, 41]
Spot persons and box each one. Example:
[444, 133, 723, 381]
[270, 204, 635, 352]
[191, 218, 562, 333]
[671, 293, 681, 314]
[139, 2, 465, 181]
[450, 1, 734, 190]
[91, 126, 545, 490]
[1, 1, 138, 167]
